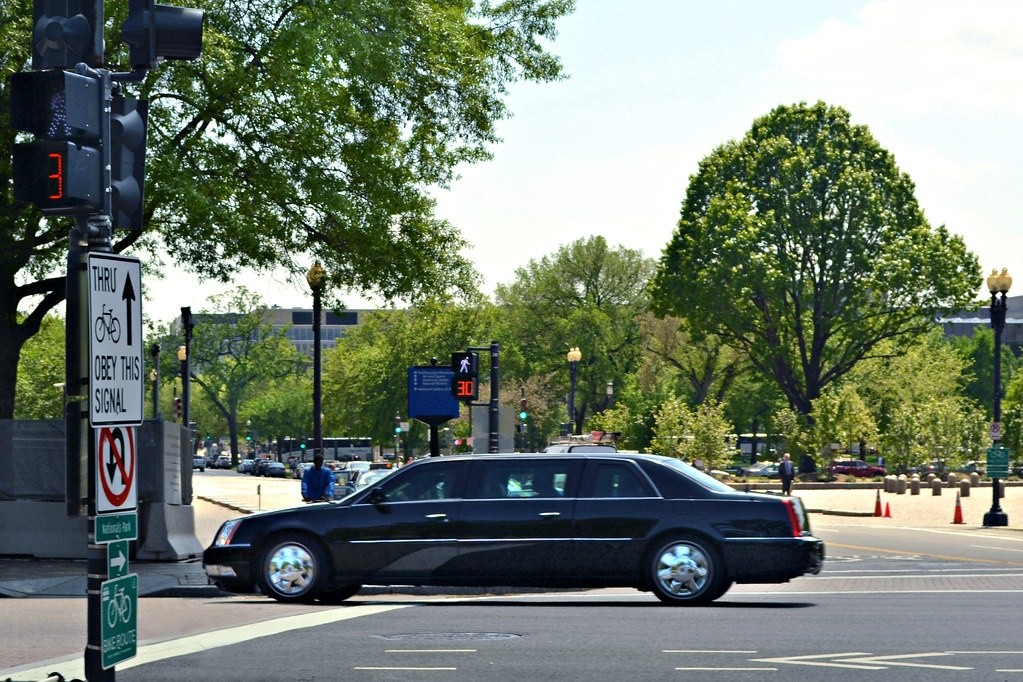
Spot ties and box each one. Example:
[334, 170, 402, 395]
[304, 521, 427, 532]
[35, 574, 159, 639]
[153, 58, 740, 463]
[787, 463, 789, 473]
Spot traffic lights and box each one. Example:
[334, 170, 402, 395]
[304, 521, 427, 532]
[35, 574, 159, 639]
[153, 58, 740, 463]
[453, 352, 478, 398]
[519, 398, 528, 421]
[173, 397, 181, 420]
[12, 71, 102, 211]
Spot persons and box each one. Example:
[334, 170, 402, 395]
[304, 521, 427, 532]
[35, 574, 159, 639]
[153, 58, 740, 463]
[301, 453, 335, 504]
[441, 467, 460, 498]
[531, 470, 562, 497]
[778, 453, 795, 495]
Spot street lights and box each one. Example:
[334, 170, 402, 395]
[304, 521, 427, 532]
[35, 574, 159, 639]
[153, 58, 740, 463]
[982, 265, 1014, 527]
[568, 346, 582, 435]
[147, 340, 163, 418]
[604, 378, 615, 411]
[176, 344, 191, 424]
[306, 258, 331, 461]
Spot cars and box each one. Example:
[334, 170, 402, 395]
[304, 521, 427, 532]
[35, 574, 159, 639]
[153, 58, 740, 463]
[204, 452, 826, 608]
[189, 436, 445, 502]
[544, 432, 1023, 479]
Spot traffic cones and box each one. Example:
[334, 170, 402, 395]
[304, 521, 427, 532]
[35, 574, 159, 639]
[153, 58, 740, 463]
[874, 487, 882, 515]
[882, 502, 892, 517]
[949, 491, 966, 524]
